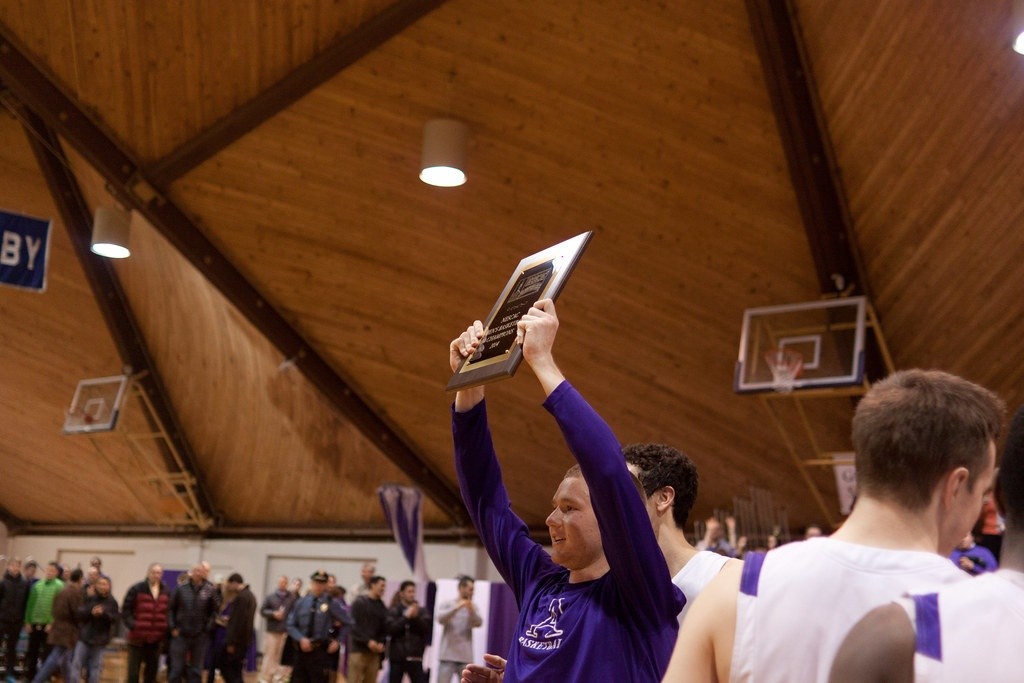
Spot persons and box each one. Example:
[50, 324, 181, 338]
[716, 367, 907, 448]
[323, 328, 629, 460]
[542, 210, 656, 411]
[827, 405, 1024, 683]
[693, 516, 822, 558]
[0, 555, 482, 683]
[448, 299, 687, 683]
[621, 442, 740, 624]
[949, 531, 997, 577]
[662, 370, 1004, 683]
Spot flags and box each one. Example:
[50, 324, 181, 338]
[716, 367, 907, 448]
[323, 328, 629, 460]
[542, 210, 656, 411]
[379, 486, 429, 582]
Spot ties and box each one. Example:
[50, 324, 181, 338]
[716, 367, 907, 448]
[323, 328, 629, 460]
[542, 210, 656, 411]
[306, 598, 318, 637]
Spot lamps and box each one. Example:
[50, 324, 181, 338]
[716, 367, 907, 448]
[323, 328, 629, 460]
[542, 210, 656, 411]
[419, 72, 469, 187]
[89, 198, 131, 258]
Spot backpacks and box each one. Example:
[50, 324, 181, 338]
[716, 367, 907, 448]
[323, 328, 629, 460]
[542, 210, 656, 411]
[52, 584, 78, 618]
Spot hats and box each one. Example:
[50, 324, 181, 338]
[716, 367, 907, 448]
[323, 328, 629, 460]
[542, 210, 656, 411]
[24, 556, 38, 566]
[310, 572, 328, 582]
